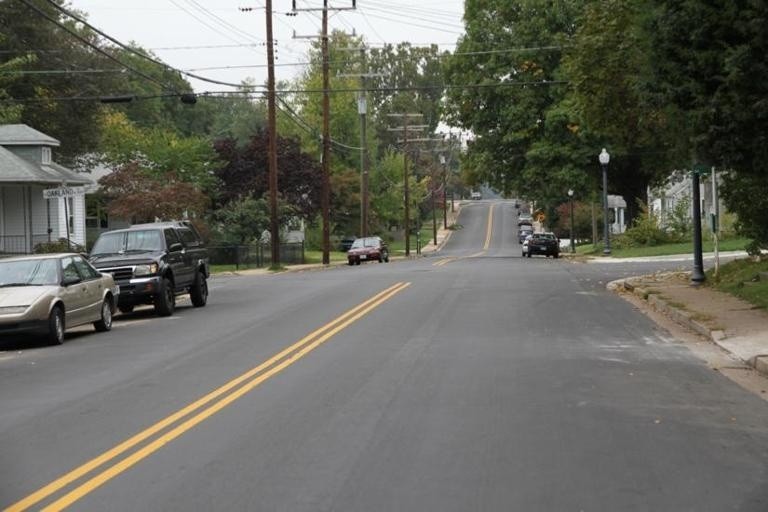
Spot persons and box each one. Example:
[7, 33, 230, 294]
[514, 200, 520, 217]
[537, 209, 544, 232]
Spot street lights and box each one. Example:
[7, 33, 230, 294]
[598, 147, 612, 254]
[568, 188, 576, 254]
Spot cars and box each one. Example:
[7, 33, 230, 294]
[515, 198, 560, 259]
[471, 192, 482, 200]
[0, 220, 210, 344]
[330, 234, 389, 266]
